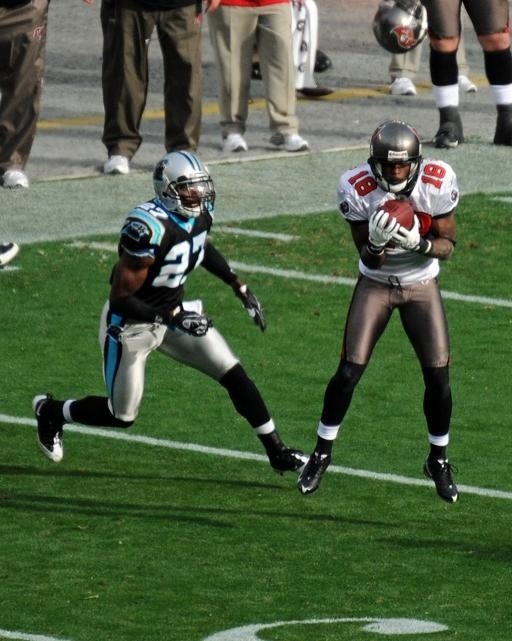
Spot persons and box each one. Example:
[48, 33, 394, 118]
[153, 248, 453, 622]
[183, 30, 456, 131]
[99, 1, 203, 174]
[0, 0, 51, 188]
[31, 149, 312, 477]
[206, 1, 332, 152]
[382, 1, 511, 150]
[298, 120, 459, 504]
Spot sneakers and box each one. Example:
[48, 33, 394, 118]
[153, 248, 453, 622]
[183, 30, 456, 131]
[423, 454, 459, 503]
[433, 122, 464, 148]
[389, 78, 416, 95]
[2, 170, 28, 189]
[266, 443, 310, 472]
[32, 393, 65, 462]
[297, 452, 331, 495]
[266, 132, 308, 152]
[103, 155, 129, 175]
[223, 132, 247, 152]
[457, 75, 477, 92]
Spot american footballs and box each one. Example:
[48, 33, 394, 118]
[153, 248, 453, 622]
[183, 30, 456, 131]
[376, 198, 413, 240]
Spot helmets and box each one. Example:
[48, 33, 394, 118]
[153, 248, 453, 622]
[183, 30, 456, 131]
[153, 150, 216, 219]
[367, 121, 422, 195]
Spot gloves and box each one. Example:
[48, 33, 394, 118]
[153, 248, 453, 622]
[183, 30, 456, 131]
[367, 210, 400, 255]
[390, 215, 427, 254]
[171, 310, 212, 337]
[236, 284, 265, 330]
